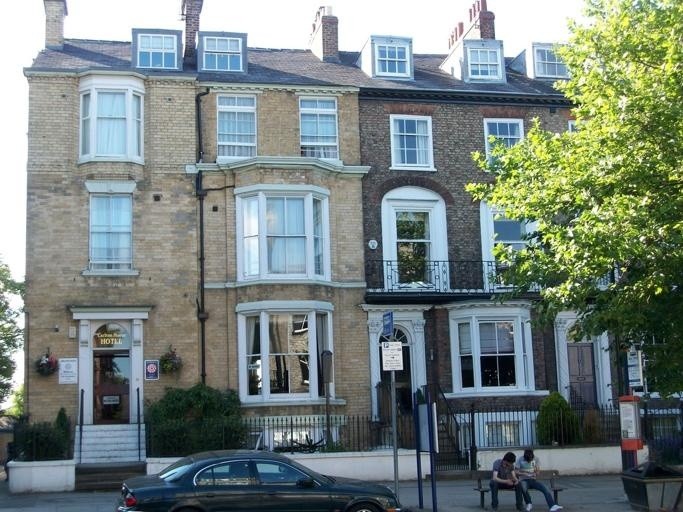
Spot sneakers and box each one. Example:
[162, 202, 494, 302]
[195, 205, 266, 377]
[549, 504, 563, 512]
[526, 504, 533, 512]
[517, 506, 527, 512]
[492, 507, 498, 512]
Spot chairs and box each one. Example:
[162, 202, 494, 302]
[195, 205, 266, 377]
[198, 462, 267, 483]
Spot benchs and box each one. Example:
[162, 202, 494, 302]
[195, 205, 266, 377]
[473, 470, 563, 509]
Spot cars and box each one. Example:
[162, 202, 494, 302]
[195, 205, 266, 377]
[114, 450, 400, 511]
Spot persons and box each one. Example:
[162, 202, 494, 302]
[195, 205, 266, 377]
[514, 447, 563, 512]
[489, 452, 526, 512]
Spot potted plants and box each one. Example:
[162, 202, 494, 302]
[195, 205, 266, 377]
[159, 344, 183, 383]
[35, 351, 58, 377]
[6, 408, 77, 493]
